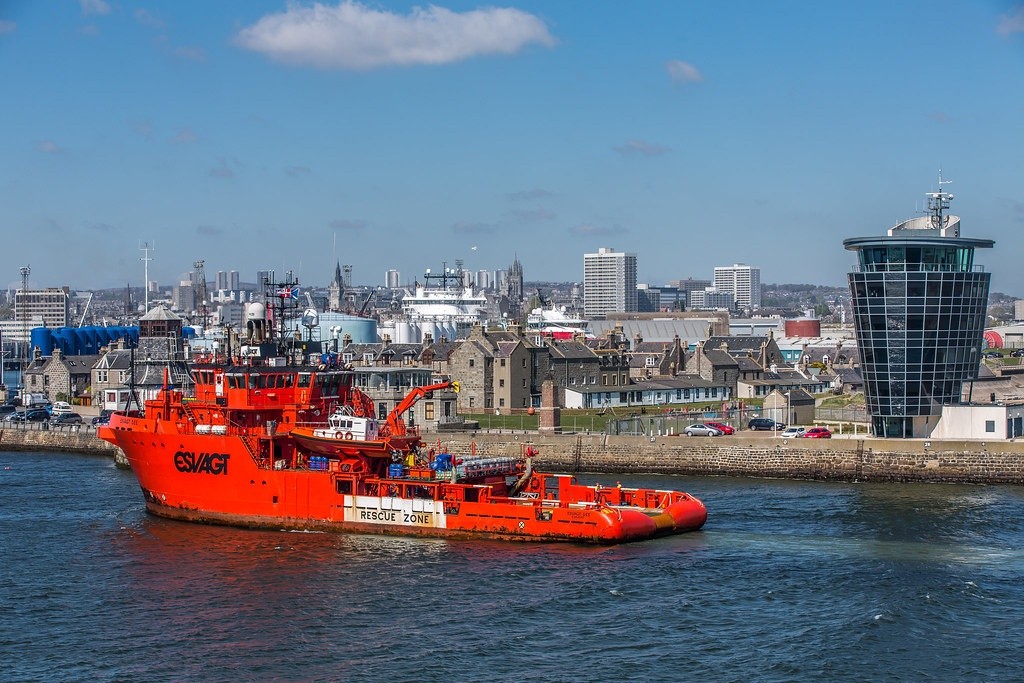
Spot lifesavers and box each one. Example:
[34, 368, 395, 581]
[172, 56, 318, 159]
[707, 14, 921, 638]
[345, 432, 353, 441]
[335, 432, 343, 440]
[530, 477, 539, 489]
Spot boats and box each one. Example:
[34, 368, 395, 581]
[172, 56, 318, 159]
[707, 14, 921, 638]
[289, 381, 460, 458]
[97, 270, 707, 545]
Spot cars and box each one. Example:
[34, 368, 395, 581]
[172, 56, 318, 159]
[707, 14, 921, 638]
[683, 423, 723, 437]
[748, 418, 786, 430]
[0, 398, 51, 423]
[101, 410, 116, 418]
[704, 422, 735, 435]
[92, 416, 109, 425]
[50, 413, 82, 428]
[987, 351, 1003, 358]
[781, 427, 806, 438]
[804, 427, 831, 438]
[1010, 349, 1024, 357]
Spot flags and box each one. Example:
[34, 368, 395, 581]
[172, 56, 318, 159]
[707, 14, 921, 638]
[277, 288, 291, 298]
[290, 289, 298, 300]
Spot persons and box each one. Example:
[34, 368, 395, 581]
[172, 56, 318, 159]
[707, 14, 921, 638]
[593, 483, 600, 503]
[415, 281, 420, 288]
[617, 481, 622, 488]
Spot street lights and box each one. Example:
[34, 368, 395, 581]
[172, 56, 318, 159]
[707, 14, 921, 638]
[1009, 413, 1019, 438]
[770, 389, 776, 437]
[784, 390, 790, 427]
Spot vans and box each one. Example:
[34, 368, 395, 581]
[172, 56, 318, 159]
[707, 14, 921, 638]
[52, 402, 73, 417]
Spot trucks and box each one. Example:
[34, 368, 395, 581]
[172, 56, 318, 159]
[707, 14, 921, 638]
[22, 393, 52, 408]
